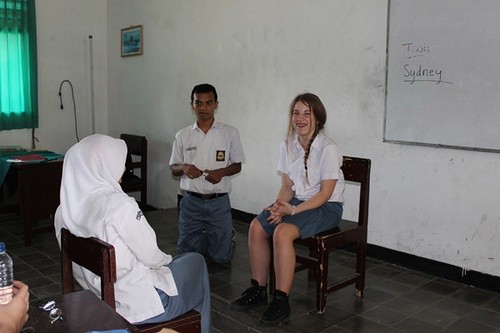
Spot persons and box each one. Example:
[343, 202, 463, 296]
[169, 83, 245, 263]
[54, 134, 211, 333]
[0, 280, 29, 333]
[231, 89, 345, 324]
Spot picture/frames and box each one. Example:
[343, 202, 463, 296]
[120, 25, 143, 57]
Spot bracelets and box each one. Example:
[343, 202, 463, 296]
[291, 206, 296, 216]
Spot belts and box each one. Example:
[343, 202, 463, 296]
[186, 190, 228, 200]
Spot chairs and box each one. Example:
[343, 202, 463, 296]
[269, 156, 371, 313]
[61, 228, 201, 333]
[120, 134, 147, 212]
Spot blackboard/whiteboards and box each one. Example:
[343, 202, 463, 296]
[382, 0, 500, 153]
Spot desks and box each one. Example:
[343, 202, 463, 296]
[0, 150, 63, 246]
[19, 290, 133, 333]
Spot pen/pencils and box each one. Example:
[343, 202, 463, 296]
[199, 170, 207, 177]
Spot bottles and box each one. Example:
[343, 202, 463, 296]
[0, 242, 14, 305]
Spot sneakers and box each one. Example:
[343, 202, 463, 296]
[261, 289, 290, 323]
[229, 278, 268, 311]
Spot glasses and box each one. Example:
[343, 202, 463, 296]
[39, 300, 63, 324]
